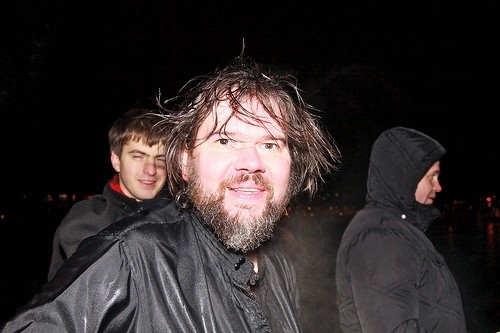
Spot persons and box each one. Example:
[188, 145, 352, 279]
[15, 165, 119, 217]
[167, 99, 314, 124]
[0, 66, 342, 333]
[48, 113, 170, 280]
[336, 128, 467, 333]
